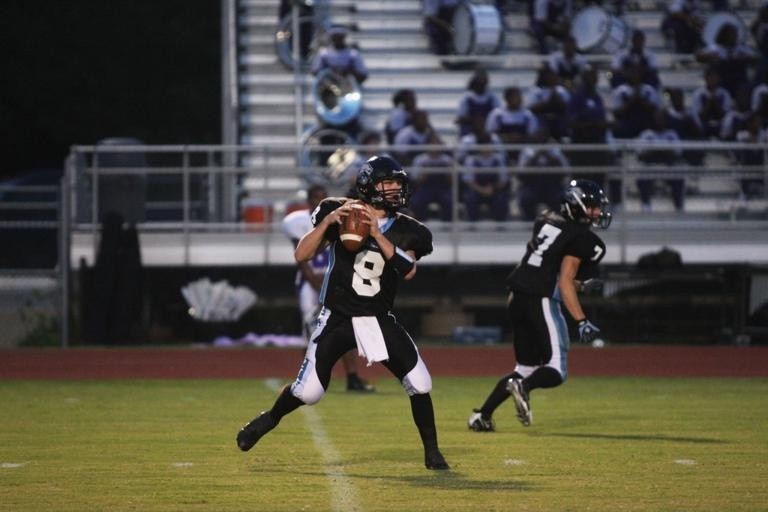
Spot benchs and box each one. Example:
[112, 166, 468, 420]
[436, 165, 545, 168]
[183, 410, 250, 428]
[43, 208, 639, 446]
[239, 0, 766, 218]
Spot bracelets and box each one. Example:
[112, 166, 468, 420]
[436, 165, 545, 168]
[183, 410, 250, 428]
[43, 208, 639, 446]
[388, 244, 414, 280]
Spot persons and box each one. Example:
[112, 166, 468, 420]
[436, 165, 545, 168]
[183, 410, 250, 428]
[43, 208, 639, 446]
[236, 151, 449, 470]
[467, 179, 613, 433]
[282, 183, 375, 393]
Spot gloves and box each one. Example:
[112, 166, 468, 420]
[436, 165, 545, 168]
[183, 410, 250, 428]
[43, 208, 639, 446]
[577, 319, 601, 344]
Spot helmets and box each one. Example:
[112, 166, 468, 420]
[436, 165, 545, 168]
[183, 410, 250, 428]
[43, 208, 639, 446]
[560, 179, 613, 231]
[355, 154, 409, 210]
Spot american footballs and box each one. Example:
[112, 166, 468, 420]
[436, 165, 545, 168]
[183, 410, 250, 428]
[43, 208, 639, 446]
[339, 199, 371, 250]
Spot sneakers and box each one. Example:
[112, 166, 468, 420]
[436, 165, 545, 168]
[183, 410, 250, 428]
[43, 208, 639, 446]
[236, 411, 279, 451]
[467, 410, 494, 431]
[507, 377, 532, 427]
[346, 373, 376, 392]
[425, 452, 449, 469]
